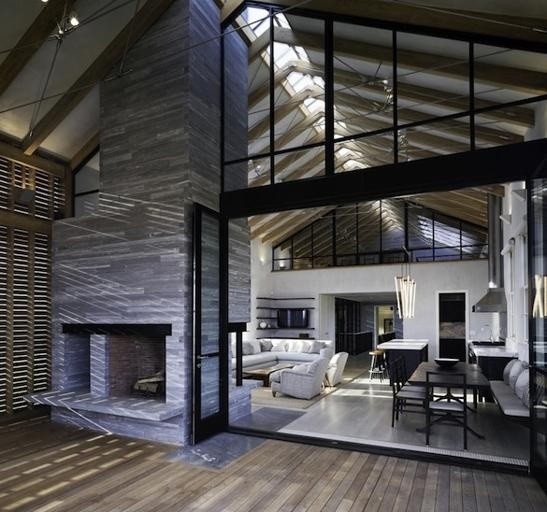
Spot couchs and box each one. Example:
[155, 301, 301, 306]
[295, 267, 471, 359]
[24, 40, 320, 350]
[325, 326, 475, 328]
[227, 329, 348, 402]
[488, 360, 546, 422]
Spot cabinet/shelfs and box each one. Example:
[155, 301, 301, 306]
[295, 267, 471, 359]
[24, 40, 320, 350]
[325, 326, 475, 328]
[341, 332, 372, 356]
[377, 335, 431, 384]
[469, 336, 518, 388]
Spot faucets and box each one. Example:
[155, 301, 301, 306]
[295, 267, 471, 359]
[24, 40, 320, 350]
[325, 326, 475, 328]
[481, 325, 492, 339]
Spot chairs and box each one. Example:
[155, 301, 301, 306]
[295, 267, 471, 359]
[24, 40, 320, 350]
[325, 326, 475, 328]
[389, 332, 492, 451]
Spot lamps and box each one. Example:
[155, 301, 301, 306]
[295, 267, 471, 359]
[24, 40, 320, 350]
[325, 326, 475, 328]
[15, 188, 35, 205]
[395, 250, 417, 319]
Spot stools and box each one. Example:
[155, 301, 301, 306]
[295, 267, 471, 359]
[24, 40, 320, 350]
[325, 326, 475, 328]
[369, 347, 388, 384]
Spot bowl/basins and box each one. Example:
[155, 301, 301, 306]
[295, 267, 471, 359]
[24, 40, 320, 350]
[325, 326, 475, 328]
[434, 358, 460, 368]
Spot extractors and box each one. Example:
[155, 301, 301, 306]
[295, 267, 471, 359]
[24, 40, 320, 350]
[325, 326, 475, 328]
[472, 288, 507, 313]
